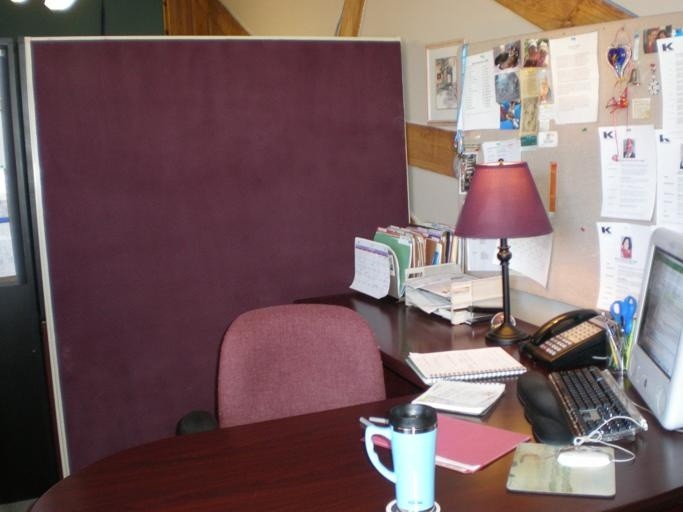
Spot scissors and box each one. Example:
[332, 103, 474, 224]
[610, 295, 637, 334]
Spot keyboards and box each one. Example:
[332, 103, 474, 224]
[516, 366, 640, 445]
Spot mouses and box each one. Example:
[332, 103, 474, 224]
[559, 451, 610, 468]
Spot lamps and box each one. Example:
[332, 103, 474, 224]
[458, 159, 554, 346]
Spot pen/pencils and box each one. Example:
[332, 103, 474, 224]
[605, 321, 635, 370]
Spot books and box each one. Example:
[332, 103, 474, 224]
[405, 344, 528, 386]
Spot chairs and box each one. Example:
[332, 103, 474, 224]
[170, 303, 386, 444]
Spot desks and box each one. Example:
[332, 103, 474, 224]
[27, 287, 682, 511]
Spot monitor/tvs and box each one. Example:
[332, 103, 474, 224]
[627, 228, 683, 432]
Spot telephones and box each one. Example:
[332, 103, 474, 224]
[524, 310, 610, 369]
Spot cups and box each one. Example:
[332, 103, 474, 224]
[362, 403, 438, 511]
